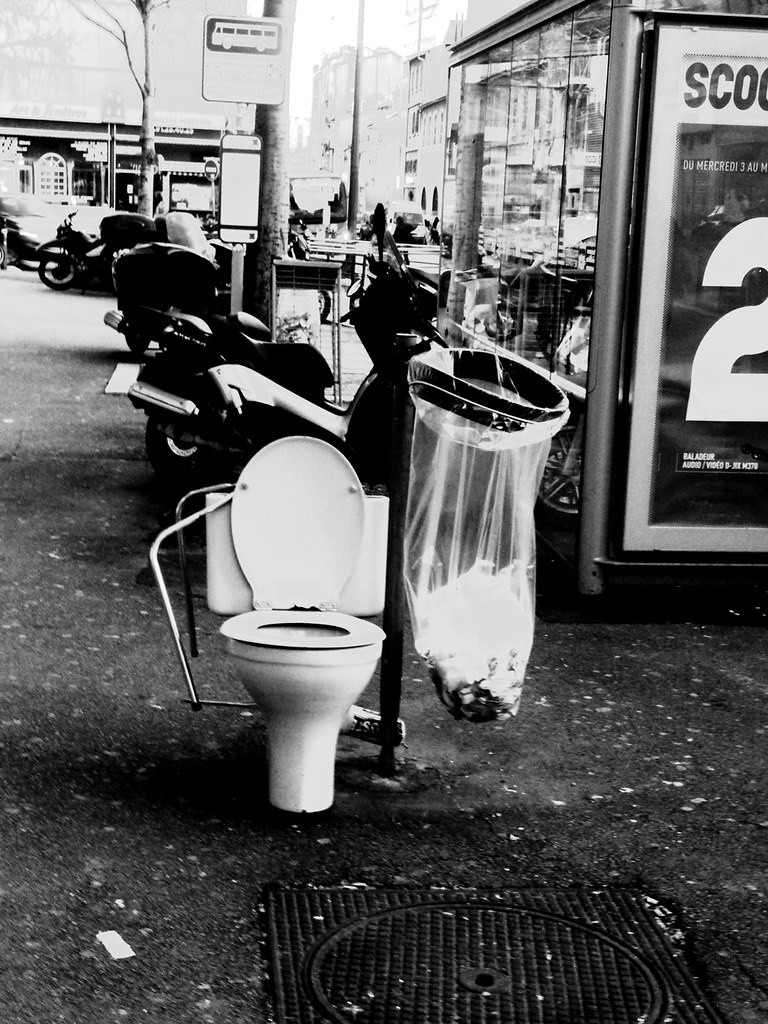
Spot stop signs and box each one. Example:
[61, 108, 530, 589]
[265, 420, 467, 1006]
[203, 160, 219, 181]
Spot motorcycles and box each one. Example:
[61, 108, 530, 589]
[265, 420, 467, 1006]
[275, 219, 331, 325]
[36, 208, 155, 292]
[108, 202, 453, 521]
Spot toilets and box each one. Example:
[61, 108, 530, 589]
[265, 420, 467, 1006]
[201, 431, 391, 821]
